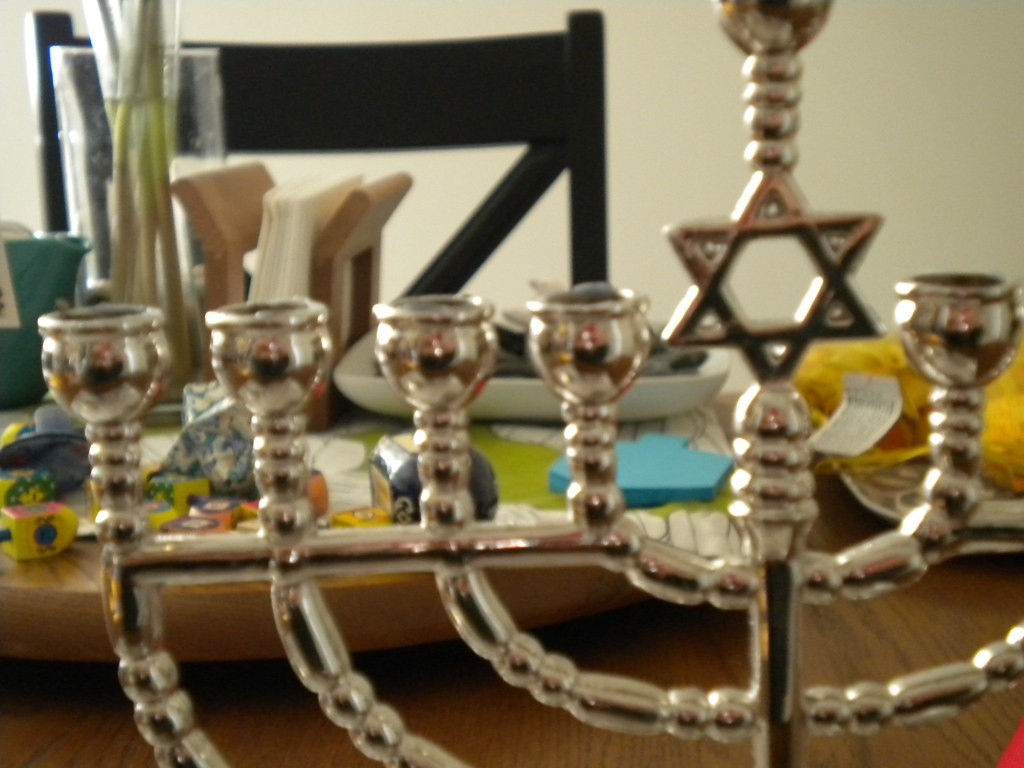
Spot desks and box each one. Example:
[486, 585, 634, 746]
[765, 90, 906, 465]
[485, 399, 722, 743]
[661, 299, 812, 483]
[0, 390, 1024, 768]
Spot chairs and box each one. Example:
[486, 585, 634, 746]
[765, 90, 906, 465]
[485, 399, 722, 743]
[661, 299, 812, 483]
[31, 5, 612, 299]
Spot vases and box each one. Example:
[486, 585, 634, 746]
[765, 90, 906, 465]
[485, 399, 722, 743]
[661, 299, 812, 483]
[47, 45, 230, 432]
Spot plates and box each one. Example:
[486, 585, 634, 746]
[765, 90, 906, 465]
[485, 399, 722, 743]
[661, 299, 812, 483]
[332, 326, 727, 420]
